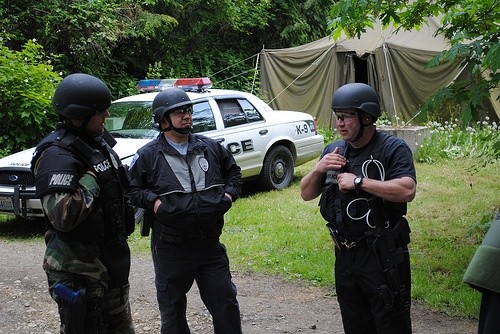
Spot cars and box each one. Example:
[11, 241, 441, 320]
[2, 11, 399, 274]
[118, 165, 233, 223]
[0, 78, 324, 226]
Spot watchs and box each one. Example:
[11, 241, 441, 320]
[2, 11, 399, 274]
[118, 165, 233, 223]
[353, 174, 365, 193]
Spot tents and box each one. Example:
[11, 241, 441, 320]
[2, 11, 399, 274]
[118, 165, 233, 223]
[260, 7, 500, 130]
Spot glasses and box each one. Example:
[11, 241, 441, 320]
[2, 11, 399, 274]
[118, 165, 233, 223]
[332, 111, 358, 119]
[169, 105, 194, 118]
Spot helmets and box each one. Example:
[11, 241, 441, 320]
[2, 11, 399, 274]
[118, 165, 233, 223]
[332, 83, 380, 118]
[52, 73, 111, 120]
[152, 87, 195, 123]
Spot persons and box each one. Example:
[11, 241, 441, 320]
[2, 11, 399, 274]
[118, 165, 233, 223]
[126, 89, 242, 334]
[300, 83, 416, 334]
[30, 73, 135, 334]
